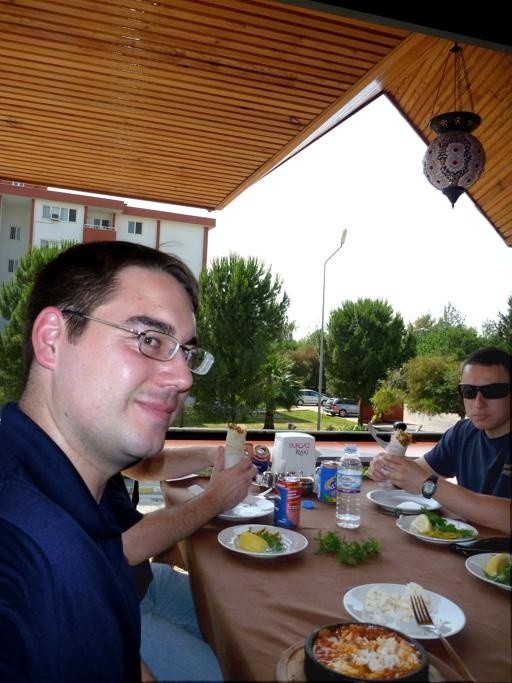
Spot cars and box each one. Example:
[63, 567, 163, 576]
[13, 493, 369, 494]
[297, 388, 328, 404]
[326, 396, 361, 417]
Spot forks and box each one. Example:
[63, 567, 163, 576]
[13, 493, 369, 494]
[409, 594, 476, 682]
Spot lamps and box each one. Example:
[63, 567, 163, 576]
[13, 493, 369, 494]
[422, 43, 488, 210]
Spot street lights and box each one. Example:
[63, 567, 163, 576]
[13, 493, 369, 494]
[316, 229, 348, 430]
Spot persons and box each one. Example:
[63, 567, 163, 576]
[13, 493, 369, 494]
[100, 442, 259, 683]
[366, 347, 512, 535]
[1, 240, 214, 683]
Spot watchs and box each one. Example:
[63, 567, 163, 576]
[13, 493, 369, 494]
[422, 474, 439, 499]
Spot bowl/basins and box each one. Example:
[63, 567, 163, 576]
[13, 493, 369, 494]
[303, 622, 429, 683]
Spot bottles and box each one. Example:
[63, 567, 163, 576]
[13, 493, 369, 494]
[336, 443, 362, 530]
[273, 477, 301, 530]
[250, 445, 271, 473]
[317, 460, 341, 504]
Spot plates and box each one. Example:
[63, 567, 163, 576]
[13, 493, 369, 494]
[216, 523, 308, 559]
[218, 495, 274, 522]
[394, 514, 478, 544]
[465, 552, 512, 591]
[342, 582, 466, 640]
[365, 488, 442, 516]
[275, 630, 463, 683]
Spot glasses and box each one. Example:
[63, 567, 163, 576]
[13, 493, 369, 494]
[58, 307, 215, 376]
[455, 382, 512, 400]
